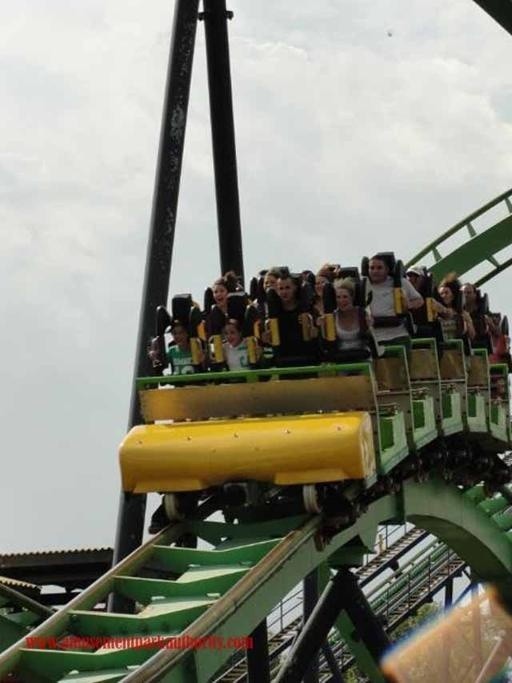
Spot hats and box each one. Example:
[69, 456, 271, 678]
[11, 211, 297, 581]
[406, 265, 425, 277]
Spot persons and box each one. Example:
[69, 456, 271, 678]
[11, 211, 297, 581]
[143, 251, 511, 391]
